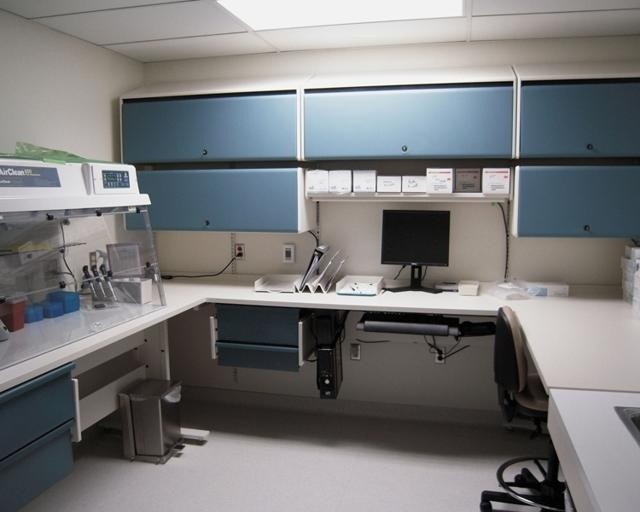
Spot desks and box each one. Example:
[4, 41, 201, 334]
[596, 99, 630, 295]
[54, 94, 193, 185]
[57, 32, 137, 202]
[0, 273, 640, 511]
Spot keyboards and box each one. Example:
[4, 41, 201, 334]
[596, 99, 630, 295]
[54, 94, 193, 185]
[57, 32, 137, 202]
[358, 312, 444, 325]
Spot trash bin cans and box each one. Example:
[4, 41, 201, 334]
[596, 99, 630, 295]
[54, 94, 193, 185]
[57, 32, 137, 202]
[118, 377, 184, 465]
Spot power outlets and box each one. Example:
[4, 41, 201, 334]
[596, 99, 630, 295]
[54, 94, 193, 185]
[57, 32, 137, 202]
[434, 348, 445, 364]
[235, 244, 244, 259]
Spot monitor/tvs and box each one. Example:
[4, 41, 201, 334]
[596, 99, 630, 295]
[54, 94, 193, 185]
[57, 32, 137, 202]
[106, 241, 144, 278]
[380, 209, 451, 294]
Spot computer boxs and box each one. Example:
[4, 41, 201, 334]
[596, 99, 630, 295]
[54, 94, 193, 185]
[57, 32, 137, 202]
[317, 336, 342, 400]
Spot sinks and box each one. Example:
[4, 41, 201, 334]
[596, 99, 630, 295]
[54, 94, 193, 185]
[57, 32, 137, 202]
[614, 405, 640, 447]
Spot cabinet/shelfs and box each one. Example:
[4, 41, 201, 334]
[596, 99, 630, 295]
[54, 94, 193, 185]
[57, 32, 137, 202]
[518, 83, 640, 237]
[304, 86, 513, 158]
[122, 95, 298, 234]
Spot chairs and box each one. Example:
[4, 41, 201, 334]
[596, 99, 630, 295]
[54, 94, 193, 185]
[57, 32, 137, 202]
[479, 306, 567, 512]
